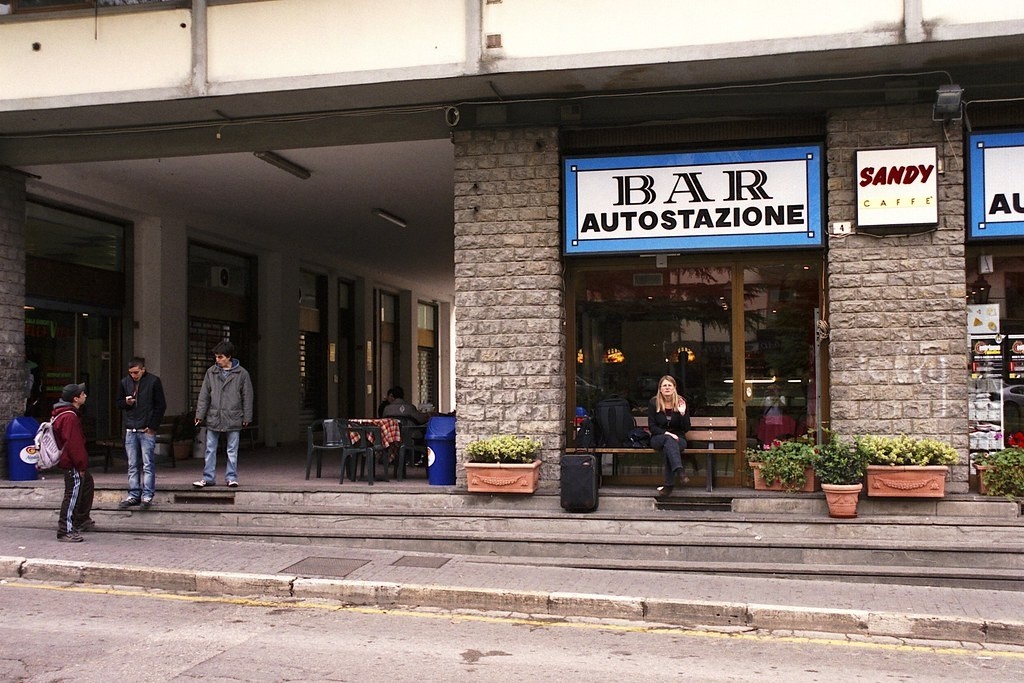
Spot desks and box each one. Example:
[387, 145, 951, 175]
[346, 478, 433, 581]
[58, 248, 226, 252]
[349, 418, 404, 481]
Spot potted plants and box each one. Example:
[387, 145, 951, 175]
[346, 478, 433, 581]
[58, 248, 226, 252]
[861, 432, 958, 497]
[814, 428, 863, 517]
[464, 434, 542, 493]
[172, 414, 201, 458]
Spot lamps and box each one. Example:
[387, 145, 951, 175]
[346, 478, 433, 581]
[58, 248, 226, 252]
[971, 277, 993, 305]
[931, 84, 964, 122]
[255, 151, 311, 180]
[375, 209, 407, 229]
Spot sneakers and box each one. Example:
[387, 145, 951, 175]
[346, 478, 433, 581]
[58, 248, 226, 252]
[72, 518, 95, 531]
[141, 495, 152, 508]
[226, 479, 239, 487]
[193, 479, 216, 487]
[56, 531, 83, 542]
[119, 496, 141, 507]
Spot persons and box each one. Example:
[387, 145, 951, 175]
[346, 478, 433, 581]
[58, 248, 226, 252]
[193, 343, 254, 487]
[647, 376, 690, 499]
[117, 357, 166, 507]
[378, 385, 427, 466]
[52, 383, 96, 542]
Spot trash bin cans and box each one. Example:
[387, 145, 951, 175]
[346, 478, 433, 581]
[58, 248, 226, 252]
[5, 417, 39, 479]
[425, 417, 455, 485]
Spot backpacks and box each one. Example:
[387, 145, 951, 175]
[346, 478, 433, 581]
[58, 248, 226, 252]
[575, 409, 607, 448]
[596, 394, 637, 448]
[36, 411, 77, 470]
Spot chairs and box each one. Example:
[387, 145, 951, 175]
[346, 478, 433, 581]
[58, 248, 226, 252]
[397, 422, 429, 483]
[334, 419, 389, 485]
[305, 418, 346, 480]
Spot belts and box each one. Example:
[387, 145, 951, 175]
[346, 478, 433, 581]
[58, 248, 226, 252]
[125, 428, 146, 432]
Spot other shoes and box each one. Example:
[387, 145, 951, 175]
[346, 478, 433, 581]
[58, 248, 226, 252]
[659, 485, 673, 497]
[677, 468, 689, 485]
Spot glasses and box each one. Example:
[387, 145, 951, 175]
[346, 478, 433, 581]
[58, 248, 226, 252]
[127, 368, 142, 374]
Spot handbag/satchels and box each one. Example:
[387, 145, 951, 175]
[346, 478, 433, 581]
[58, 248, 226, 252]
[622, 427, 651, 448]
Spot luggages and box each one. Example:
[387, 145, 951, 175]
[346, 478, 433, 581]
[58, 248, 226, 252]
[559, 449, 599, 513]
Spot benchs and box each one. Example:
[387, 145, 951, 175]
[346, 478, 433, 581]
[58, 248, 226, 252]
[93, 415, 179, 472]
[565, 415, 737, 492]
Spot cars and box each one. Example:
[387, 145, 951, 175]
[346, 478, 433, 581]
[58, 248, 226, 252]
[985, 378, 1024, 416]
[576, 376, 603, 391]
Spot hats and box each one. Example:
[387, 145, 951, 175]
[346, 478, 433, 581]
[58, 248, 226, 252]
[63, 383, 85, 402]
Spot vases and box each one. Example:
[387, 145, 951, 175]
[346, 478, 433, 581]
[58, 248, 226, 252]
[749, 462, 820, 492]
[973, 463, 1023, 495]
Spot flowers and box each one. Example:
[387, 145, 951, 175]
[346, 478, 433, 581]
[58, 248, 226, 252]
[747, 438, 819, 492]
[974, 433, 1024, 500]
